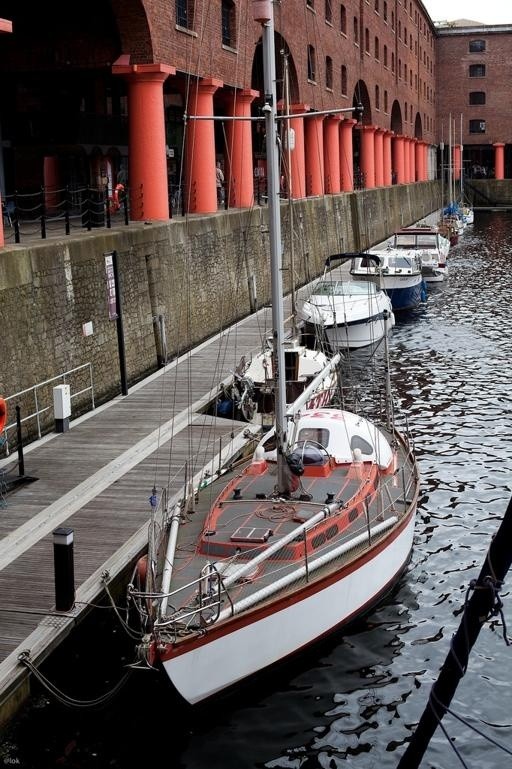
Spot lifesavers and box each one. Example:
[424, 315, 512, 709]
[0, 398, 7, 438]
[114, 184, 124, 210]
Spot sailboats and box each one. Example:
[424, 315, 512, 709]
[121, 2, 422, 711]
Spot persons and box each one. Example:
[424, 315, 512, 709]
[472, 160, 494, 179]
[97, 170, 109, 203]
[215, 160, 226, 206]
